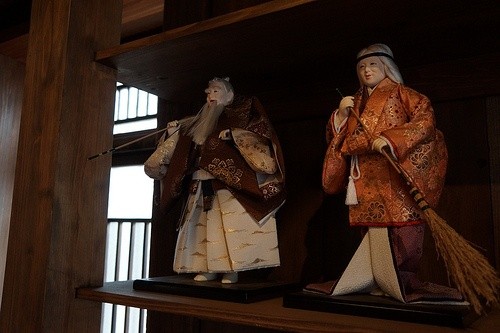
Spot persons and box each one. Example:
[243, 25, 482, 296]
[144, 75, 290, 284]
[302, 42, 465, 302]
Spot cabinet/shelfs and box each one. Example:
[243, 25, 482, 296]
[1, 0, 499, 333]
